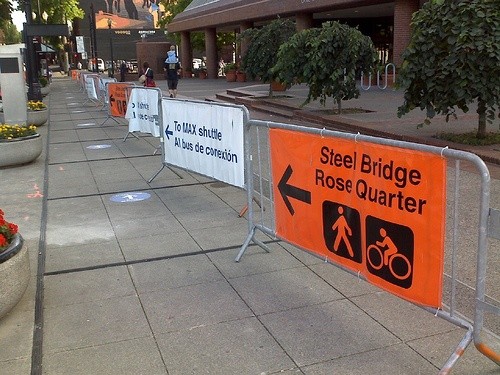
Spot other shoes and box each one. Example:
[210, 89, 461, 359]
[170, 94, 174, 98]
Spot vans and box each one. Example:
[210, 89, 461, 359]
[87, 57, 105, 74]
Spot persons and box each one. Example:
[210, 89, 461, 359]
[143, 62, 152, 87]
[77, 61, 82, 69]
[163, 61, 181, 98]
[139, 68, 156, 87]
[119, 61, 129, 82]
[164, 45, 177, 63]
[218, 58, 226, 76]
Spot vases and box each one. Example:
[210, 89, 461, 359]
[0, 209, 19, 252]
[0, 110, 49, 127]
[0, 232, 31, 319]
[0, 134, 43, 167]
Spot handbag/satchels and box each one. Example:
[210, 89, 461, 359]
[138, 68, 150, 84]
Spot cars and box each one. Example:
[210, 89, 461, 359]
[192, 58, 205, 70]
[104, 59, 138, 73]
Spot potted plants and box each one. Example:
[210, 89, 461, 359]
[224, 63, 246, 82]
[194, 68, 206, 79]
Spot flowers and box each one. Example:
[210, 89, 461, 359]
[27, 100, 48, 110]
[0, 120, 37, 140]
